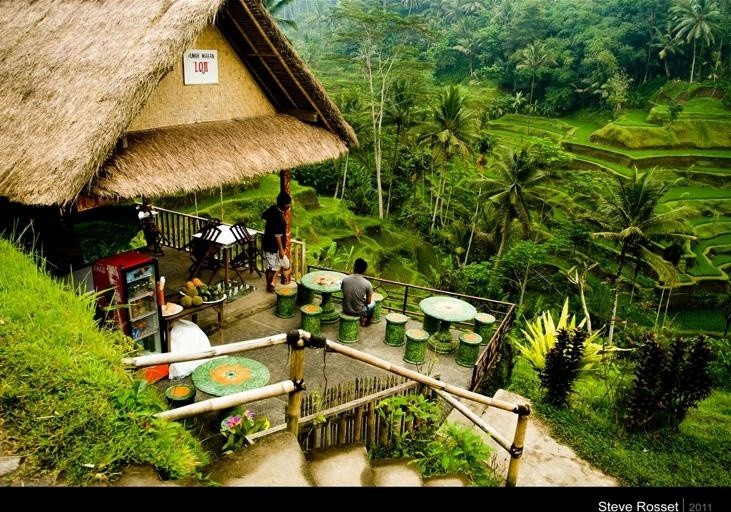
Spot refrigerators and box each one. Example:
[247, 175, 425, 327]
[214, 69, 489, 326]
[92, 250, 168, 357]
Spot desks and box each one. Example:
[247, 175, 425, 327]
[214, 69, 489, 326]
[160, 291, 223, 351]
[191, 224, 258, 287]
[137, 209, 158, 220]
[191, 356, 270, 434]
[419, 296, 477, 353]
[300, 272, 349, 323]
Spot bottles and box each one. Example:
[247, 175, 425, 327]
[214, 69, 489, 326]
[129, 277, 155, 352]
[133, 266, 149, 278]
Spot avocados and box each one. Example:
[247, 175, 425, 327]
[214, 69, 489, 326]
[197, 285, 223, 301]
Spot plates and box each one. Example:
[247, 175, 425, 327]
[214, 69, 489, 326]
[180, 291, 227, 304]
[162, 304, 183, 316]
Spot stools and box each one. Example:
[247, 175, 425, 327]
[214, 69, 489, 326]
[363, 292, 385, 324]
[165, 383, 197, 429]
[295, 279, 316, 304]
[276, 287, 296, 318]
[336, 310, 361, 343]
[300, 305, 322, 337]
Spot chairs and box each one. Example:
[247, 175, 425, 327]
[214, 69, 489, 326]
[186, 225, 223, 285]
[230, 223, 266, 286]
[177, 219, 221, 260]
[133, 204, 172, 257]
[385, 312, 496, 368]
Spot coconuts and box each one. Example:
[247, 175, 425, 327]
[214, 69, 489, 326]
[179, 288, 203, 307]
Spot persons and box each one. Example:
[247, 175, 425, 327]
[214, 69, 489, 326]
[342, 258, 375, 326]
[262, 192, 291, 293]
